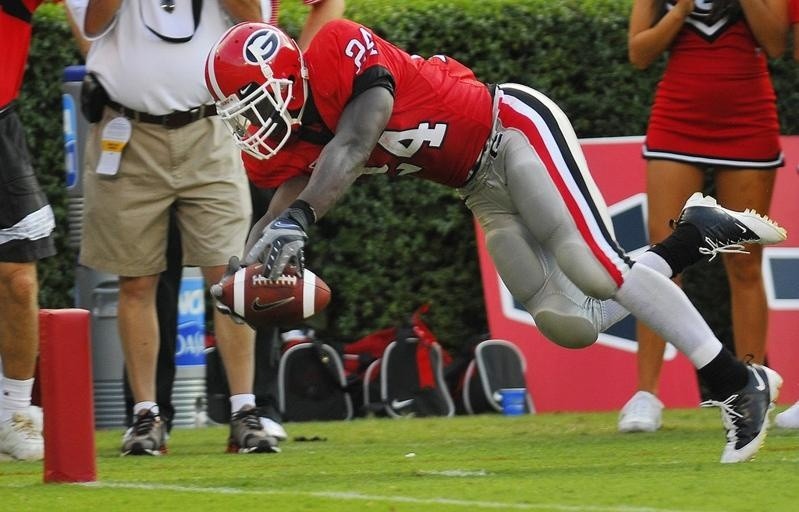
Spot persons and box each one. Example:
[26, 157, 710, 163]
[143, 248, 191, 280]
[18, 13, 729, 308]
[64, 0, 280, 457]
[204, 20, 786, 465]
[121, 0, 348, 440]
[617, 0, 788, 433]
[772, 1, 799, 430]
[0, 1, 57, 463]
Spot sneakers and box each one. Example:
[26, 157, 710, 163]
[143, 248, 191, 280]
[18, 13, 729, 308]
[617, 391, 665, 433]
[699, 353, 783, 464]
[668, 191, 788, 262]
[121, 403, 287, 456]
[774, 400, 799, 429]
[0, 411, 45, 461]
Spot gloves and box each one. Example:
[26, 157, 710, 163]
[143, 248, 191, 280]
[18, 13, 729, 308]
[245, 208, 310, 279]
[210, 256, 245, 325]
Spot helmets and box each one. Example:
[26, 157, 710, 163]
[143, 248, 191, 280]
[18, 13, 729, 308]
[205, 21, 309, 160]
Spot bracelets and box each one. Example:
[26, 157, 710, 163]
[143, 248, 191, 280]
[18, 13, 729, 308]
[290, 200, 320, 230]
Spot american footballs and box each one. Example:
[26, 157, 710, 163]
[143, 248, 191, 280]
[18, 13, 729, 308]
[210, 263, 331, 327]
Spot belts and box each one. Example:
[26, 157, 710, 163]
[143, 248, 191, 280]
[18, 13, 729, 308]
[107, 101, 217, 129]
